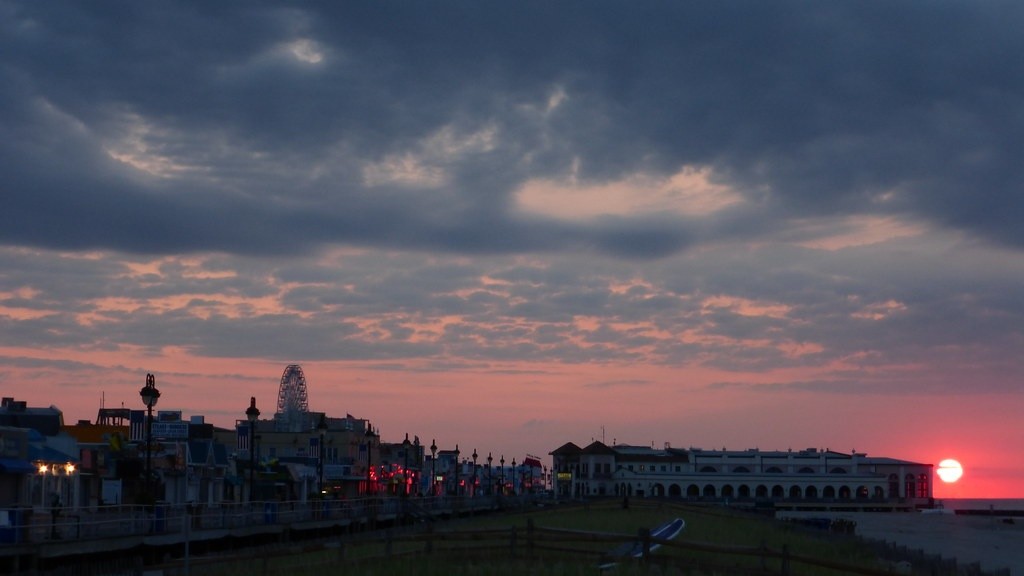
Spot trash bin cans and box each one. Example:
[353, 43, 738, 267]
[186, 500, 204, 529]
[150, 498, 169, 532]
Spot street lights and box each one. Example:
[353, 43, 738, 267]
[521, 460, 526, 490]
[487, 452, 494, 493]
[316, 414, 329, 498]
[511, 457, 517, 493]
[401, 432, 412, 497]
[543, 465, 548, 491]
[470, 448, 480, 495]
[364, 423, 377, 498]
[138, 373, 162, 497]
[550, 466, 553, 491]
[500, 454, 505, 495]
[454, 444, 461, 496]
[245, 396, 261, 501]
[430, 439, 438, 496]
[530, 463, 533, 486]
